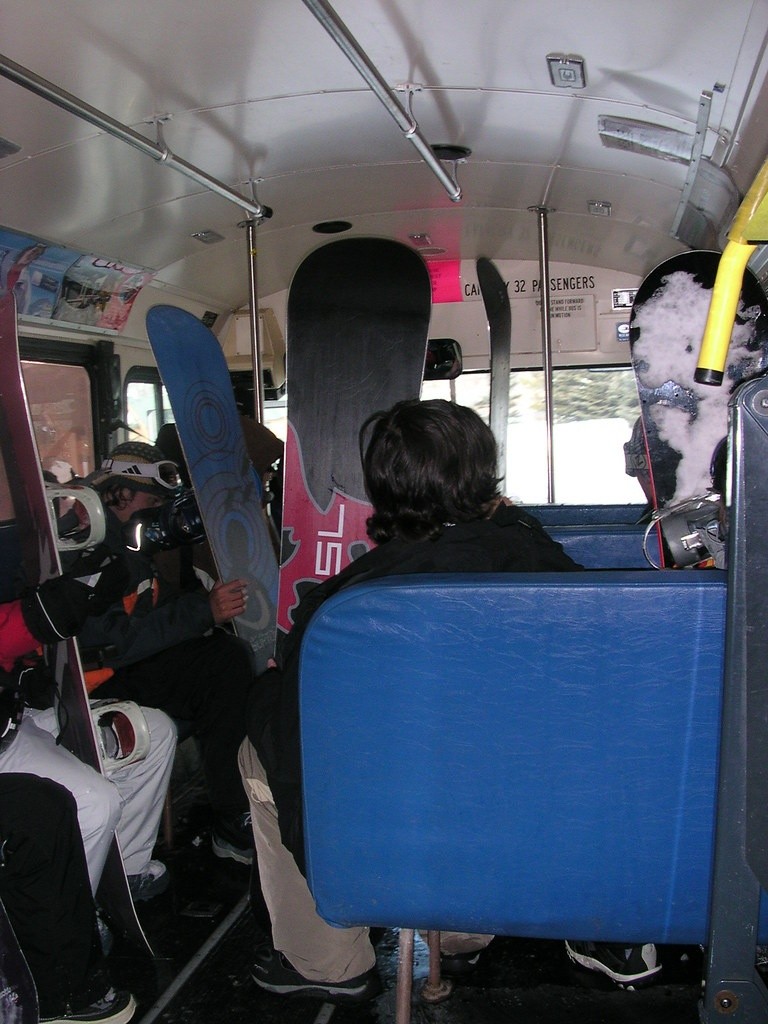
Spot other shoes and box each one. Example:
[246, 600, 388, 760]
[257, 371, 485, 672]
[244, 934, 370, 995]
[564, 939, 663, 982]
[211, 831, 254, 865]
[34, 986, 136, 1024]
[128, 871, 181, 902]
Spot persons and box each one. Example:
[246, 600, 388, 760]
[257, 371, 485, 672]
[237, 400, 665, 995]
[0, 417, 285, 1024]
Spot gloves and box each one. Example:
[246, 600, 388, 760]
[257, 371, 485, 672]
[21, 575, 104, 644]
[9, 664, 59, 711]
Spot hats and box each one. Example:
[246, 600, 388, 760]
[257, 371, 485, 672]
[47, 460, 75, 484]
[77, 441, 185, 499]
[624, 415, 680, 470]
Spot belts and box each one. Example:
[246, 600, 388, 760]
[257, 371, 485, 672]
[0, 704, 23, 756]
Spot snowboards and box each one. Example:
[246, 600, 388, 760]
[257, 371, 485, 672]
[272, 235, 434, 677]
[472, 252, 514, 506]
[625, 247, 768, 572]
[143, 301, 280, 678]
[0, 896, 41, 1024]
[0, 288, 162, 962]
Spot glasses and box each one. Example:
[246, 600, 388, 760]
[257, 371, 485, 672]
[99, 459, 183, 489]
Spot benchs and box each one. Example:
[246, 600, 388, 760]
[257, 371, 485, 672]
[299, 568, 731, 1024]
